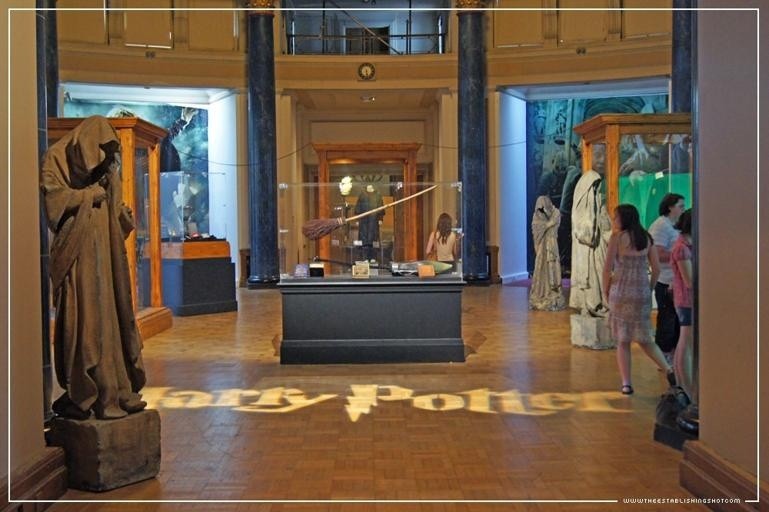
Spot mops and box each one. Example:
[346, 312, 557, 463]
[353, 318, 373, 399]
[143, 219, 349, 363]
[301, 185, 437, 242]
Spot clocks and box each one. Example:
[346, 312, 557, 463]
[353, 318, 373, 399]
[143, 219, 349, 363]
[356, 62, 377, 81]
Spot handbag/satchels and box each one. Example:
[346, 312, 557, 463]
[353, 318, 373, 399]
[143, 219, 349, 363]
[425, 231, 438, 261]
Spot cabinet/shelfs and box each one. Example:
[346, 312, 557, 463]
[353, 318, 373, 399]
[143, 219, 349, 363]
[573, 112, 695, 333]
[315, 143, 422, 277]
[47, 116, 172, 345]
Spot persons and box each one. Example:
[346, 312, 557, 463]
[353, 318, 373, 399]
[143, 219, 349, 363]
[352, 183, 387, 266]
[102, 102, 202, 244]
[39, 110, 153, 421]
[601, 204, 676, 395]
[425, 212, 459, 276]
[648, 193, 686, 372]
[527, 134, 691, 312]
[669, 207, 691, 401]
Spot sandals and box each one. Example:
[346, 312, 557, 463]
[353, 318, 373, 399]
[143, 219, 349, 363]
[621, 384, 634, 394]
[666, 368, 676, 386]
